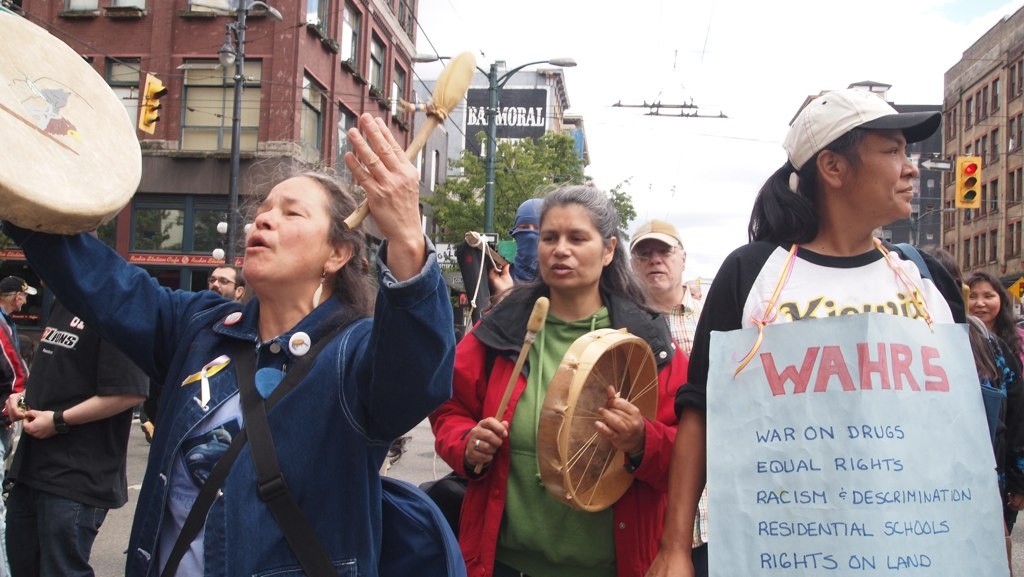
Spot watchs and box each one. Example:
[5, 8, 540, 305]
[54, 409, 73, 433]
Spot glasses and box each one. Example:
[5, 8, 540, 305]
[207, 277, 240, 286]
[637, 246, 683, 262]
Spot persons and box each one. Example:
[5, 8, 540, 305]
[0, 89, 1024, 577]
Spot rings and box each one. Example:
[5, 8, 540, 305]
[475, 439, 481, 451]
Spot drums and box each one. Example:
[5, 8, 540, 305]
[0, 4, 144, 236]
[536, 327, 659, 514]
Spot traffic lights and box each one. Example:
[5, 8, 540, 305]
[458, 293, 469, 306]
[139, 73, 169, 135]
[955, 156, 982, 209]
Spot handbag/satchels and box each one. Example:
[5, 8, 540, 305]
[978, 381, 1002, 449]
[419, 471, 466, 540]
[380, 476, 467, 577]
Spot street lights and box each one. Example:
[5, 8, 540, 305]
[191, 0, 282, 263]
[412, 54, 577, 233]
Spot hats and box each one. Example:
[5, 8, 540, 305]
[784, 89, 941, 174]
[0, 276, 38, 295]
[630, 222, 684, 253]
